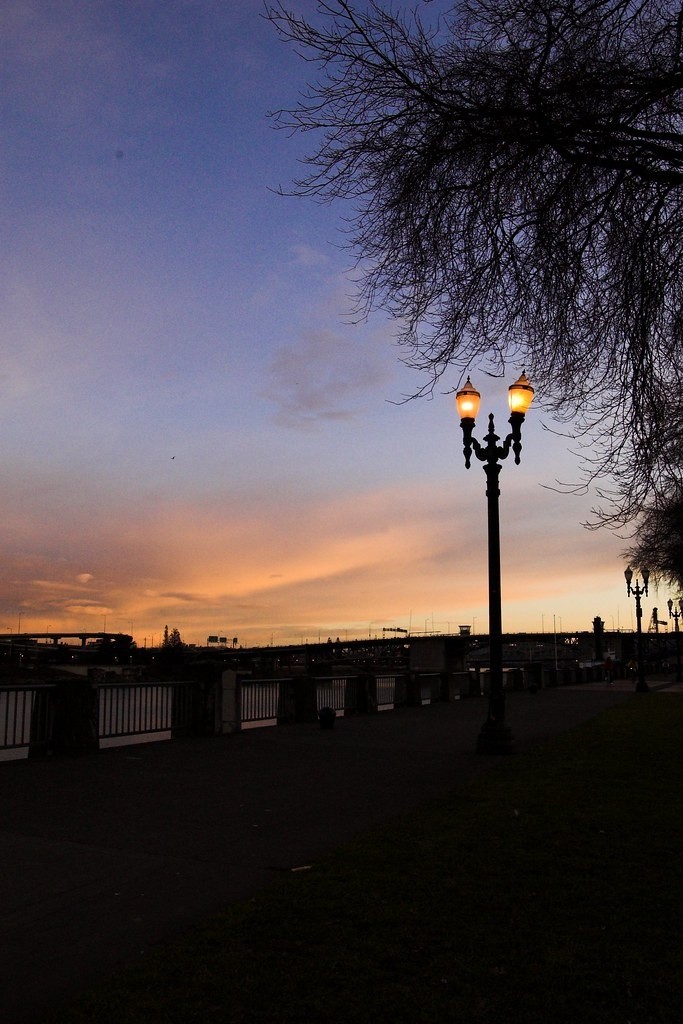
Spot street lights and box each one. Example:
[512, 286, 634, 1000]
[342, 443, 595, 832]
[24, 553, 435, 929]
[457, 365, 534, 751]
[668, 598, 683, 682]
[625, 566, 650, 692]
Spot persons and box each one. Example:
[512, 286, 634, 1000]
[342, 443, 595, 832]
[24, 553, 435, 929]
[662, 660, 669, 678]
[604, 657, 615, 686]
[624, 655, 639, 684]
